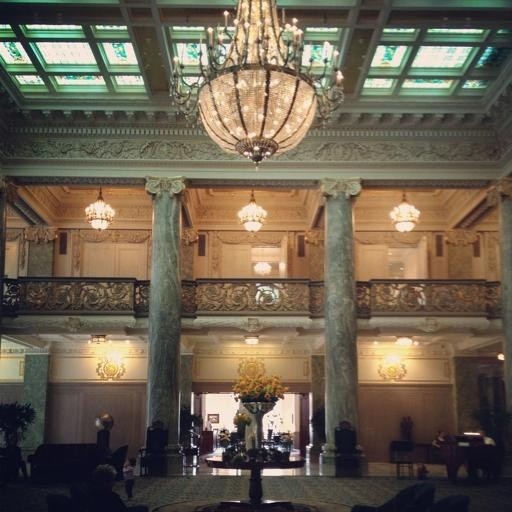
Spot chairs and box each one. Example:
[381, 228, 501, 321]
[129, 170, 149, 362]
[105, 444, 128, 481]
[351, 482, 436, 511]
[424, 494, 471, 512]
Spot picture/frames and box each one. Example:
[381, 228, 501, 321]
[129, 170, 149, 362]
[208, 414, 219, 422]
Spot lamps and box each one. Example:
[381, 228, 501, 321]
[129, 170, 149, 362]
[237, 188, 267, 233]
[254, 262, 273, 277]
[169, 0, 346, 165]
[389, 192, 421, 233]
[85, 187, 115, 231]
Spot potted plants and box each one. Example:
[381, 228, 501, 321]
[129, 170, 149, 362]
[0, 400, 36, 481]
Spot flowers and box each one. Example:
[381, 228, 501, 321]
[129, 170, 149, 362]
[233, 411, 252, 427]
[233, 374, 290, 403]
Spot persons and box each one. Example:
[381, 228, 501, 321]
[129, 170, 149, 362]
[72, 464, 152, 511]
[432, 429, 449, 448]
[122, 456, 138, 502]
[477, 426, 497, 447]
[267, 420, 276, 442]
